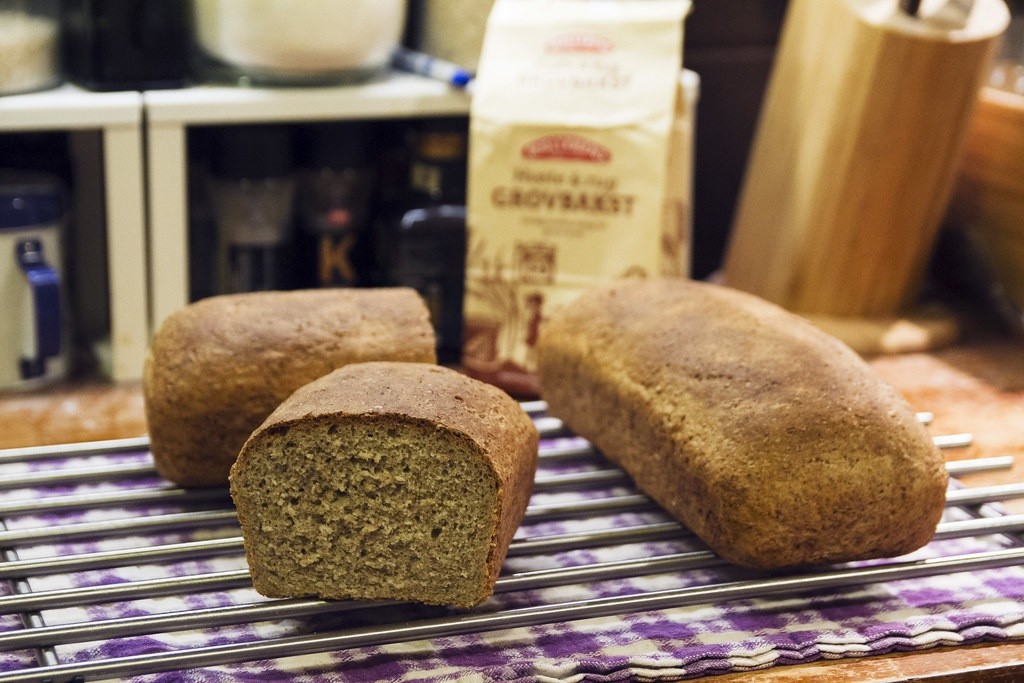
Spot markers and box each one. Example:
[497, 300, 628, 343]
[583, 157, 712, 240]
[396, 46, 475, 86]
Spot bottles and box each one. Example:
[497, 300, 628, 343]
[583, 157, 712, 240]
[194, 122, 462, 367]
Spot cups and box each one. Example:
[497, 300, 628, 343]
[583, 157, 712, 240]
[1, 166, 75, 393]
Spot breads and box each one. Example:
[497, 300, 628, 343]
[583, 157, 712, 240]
[144, 286, 437, 493]
[538, 277, 948, 565]
[228, 360, 537, 603]
